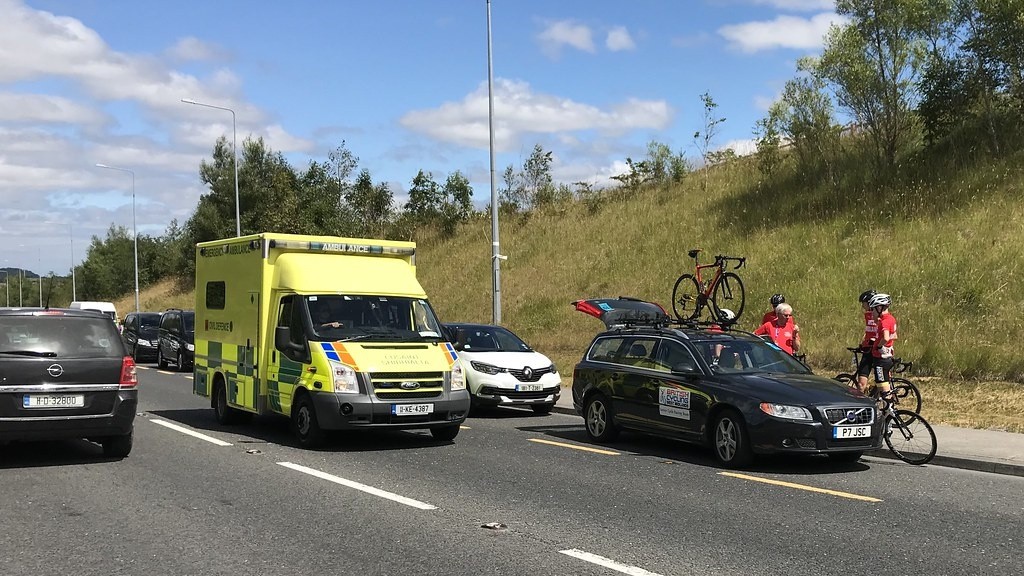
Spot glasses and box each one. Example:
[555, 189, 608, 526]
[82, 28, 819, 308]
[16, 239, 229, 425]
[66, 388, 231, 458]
[318, 309, 330, 313]
[772, 304, 778, 307]
[779, 314, 791, 317]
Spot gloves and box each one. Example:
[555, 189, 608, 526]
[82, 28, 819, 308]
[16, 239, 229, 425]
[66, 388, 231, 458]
[876, 345, 888, 354]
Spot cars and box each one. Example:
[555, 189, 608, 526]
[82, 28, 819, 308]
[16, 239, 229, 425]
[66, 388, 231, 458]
[124, 313, 161, 362]
[441, 323, 561, 414]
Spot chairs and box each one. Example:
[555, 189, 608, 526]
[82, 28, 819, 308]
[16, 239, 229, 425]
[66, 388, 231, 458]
[629, 344, 647, 366]
[716, 347, 737, 369]
[668, 344, 695, 368]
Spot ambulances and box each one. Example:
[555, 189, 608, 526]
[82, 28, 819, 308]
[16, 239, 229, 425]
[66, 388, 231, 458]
[193, 233, 471, 446]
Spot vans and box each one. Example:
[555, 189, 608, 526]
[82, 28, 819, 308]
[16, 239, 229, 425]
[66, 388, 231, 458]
[70, 301, 117, 333]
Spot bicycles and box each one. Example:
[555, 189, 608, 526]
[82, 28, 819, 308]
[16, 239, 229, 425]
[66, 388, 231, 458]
[874, 388, 937, 464]
[672, 249, 746, 325]
[834, 347, 921, 428]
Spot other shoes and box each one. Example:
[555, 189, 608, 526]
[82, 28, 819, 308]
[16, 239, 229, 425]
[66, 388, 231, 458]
[883, 431, 893, 439]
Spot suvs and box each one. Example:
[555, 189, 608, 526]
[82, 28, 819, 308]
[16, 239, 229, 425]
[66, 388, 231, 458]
[158, 308, 194, 371]
[0, 276, 137, 458]
[571, 296, 885, 470]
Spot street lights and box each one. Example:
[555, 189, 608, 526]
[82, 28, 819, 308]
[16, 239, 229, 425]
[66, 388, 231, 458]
[95, 163, 139, 313]
[18, 244, 42, 307]
[43, 220, 76, 301]
[180, 99, 241, 235]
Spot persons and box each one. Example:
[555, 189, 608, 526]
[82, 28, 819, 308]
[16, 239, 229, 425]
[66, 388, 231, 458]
[752, 303, 801, 357]
[868, 293, 898, 438]
[762, 294, 785, 325]
[857, 290, 894, 394]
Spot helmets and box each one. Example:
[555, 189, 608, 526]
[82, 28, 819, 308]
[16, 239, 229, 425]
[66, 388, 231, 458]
[867, 293, 892, 308]
[770, 293, 785, 304]
[859, 289, 877, 302]
